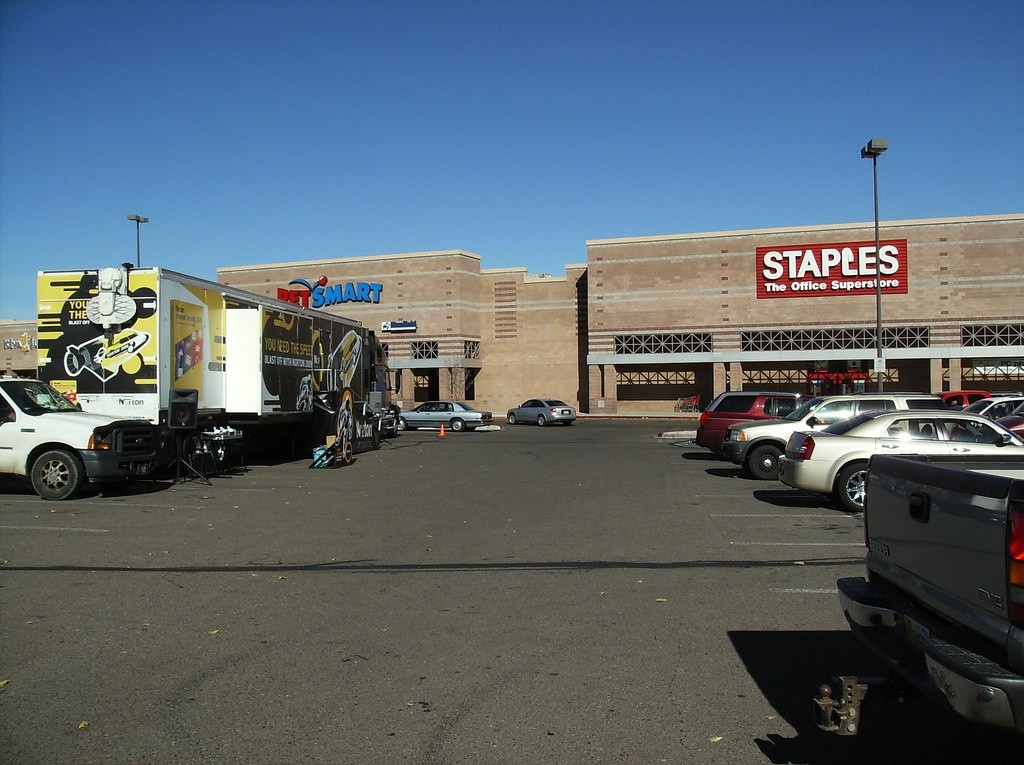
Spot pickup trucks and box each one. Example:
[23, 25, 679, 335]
[833, 452, 1024, 746]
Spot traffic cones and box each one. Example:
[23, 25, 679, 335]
[438, 423, 446, 439]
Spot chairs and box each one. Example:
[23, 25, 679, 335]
[921, 424, 933, 438]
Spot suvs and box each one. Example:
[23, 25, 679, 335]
[720, 391, 966, 482]
[694, 390, 841, 457]
[0, 377, 157, 500]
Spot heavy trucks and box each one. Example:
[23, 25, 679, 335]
[36, 266, 400, 476]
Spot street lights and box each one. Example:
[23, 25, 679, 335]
[126, 214, 149, 267]
[860, 137, 890, 395]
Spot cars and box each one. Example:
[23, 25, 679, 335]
[776, 408, 1024, 512]
[934, 389, 1024, 444]
[506, 398, 577, 427]
[397, 400, 494, 432]
